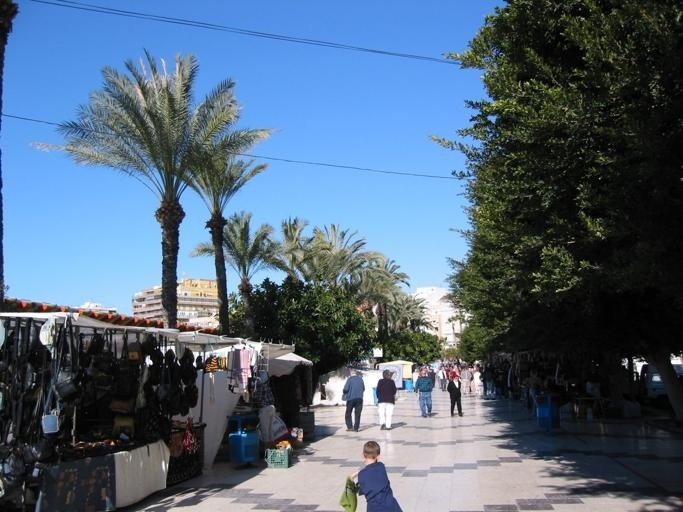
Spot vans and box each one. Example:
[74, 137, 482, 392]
[641, 364, 683, 407]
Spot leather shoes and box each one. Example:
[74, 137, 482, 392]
[346, 424, 393, 431]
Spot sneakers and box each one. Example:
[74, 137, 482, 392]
[420, 412, 462, 417]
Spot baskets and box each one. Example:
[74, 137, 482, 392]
[266, 449, 289, 469]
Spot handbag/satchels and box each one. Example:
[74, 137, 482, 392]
[0, 317, 198, 490]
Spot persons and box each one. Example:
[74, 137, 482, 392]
[349, 440, 402, 512]
[412, 356, 570, 430]
[376, 369, 397, 431]
[343, 371, 365, 432]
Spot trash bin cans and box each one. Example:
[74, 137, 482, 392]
[373, 387, 377, 404]
[403, 378, 412, 389]
[227, 415, 259, 469]
